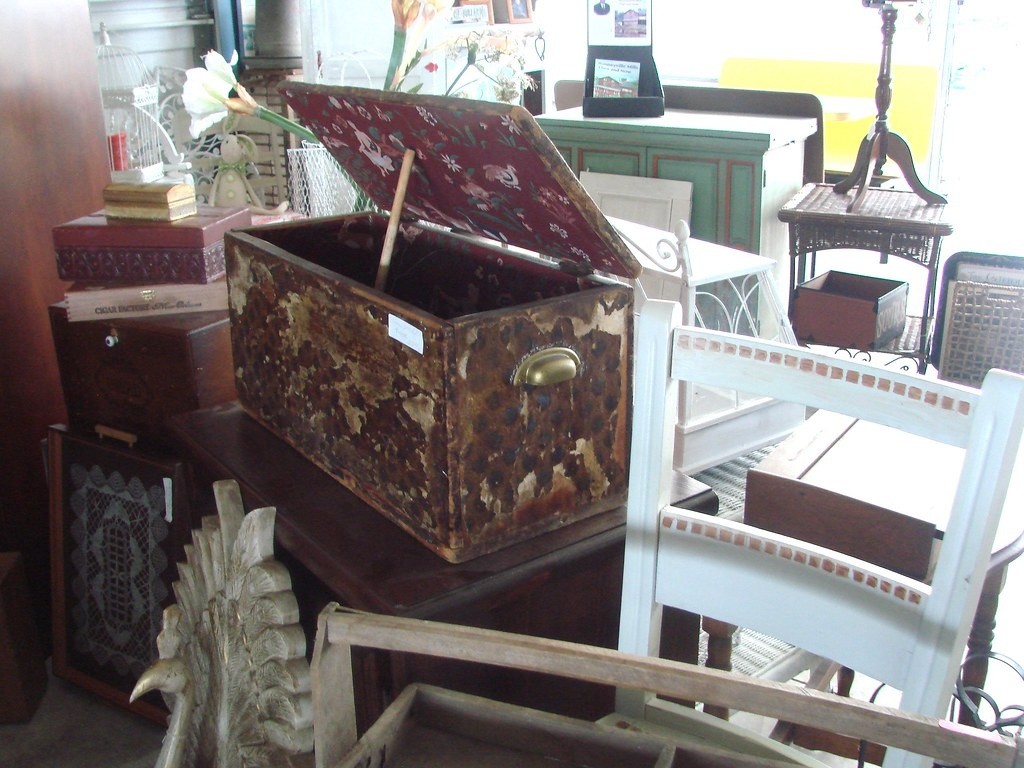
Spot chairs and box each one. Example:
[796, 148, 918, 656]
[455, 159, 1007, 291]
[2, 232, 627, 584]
[596, 300, 1024, 768]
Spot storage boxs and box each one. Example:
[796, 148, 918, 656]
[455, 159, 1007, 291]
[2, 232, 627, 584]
[796, 270, 908, 350]
[162, 401, 626, 722]
[49, 282, 237, 448]
[224, 80, 633, 564]
[744, 409, 962, 580]
[54, 207, 251, 283]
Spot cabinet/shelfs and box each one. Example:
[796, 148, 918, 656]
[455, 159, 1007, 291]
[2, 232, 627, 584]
[779, 181, 952, 376]
[534, 104, 817, 337]
